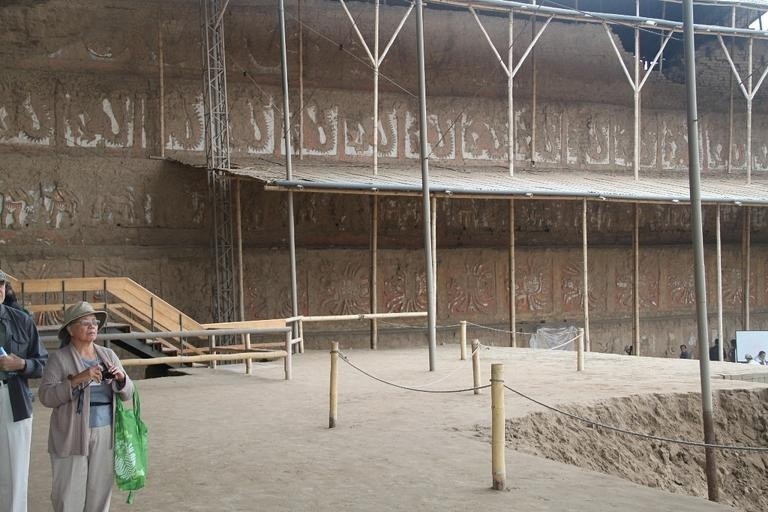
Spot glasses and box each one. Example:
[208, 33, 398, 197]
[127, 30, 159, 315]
[70, 319, 100, 326]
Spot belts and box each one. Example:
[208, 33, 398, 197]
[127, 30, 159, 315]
[0, 379, 7, 386]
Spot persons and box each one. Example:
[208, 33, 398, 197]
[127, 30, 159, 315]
[4, 274, 29, 314]
[0, 266, 48, 511]
[708, 332, 768, 367]
[679, 344, 689, 359]
[626, 345, 632, 354]
[37, 301, 147, 511]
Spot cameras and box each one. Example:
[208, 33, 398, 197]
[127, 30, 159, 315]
[100, 369, 116, 382]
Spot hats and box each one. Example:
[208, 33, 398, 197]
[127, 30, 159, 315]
[58, 301, 109, 341]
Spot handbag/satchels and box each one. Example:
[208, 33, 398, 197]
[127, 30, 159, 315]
[114, 408, 148, 491]
